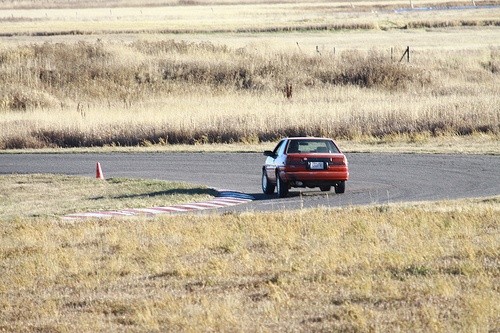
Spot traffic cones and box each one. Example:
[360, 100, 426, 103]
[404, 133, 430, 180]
[95, 162, 103, 178]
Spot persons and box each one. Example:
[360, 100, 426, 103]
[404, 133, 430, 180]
[290, 141, 299, 150]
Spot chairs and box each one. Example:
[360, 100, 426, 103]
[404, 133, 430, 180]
[288, 142, 301, 153]
[317, 147, 328, 153]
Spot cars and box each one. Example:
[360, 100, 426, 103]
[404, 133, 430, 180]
[259, 135, 349, 197]
